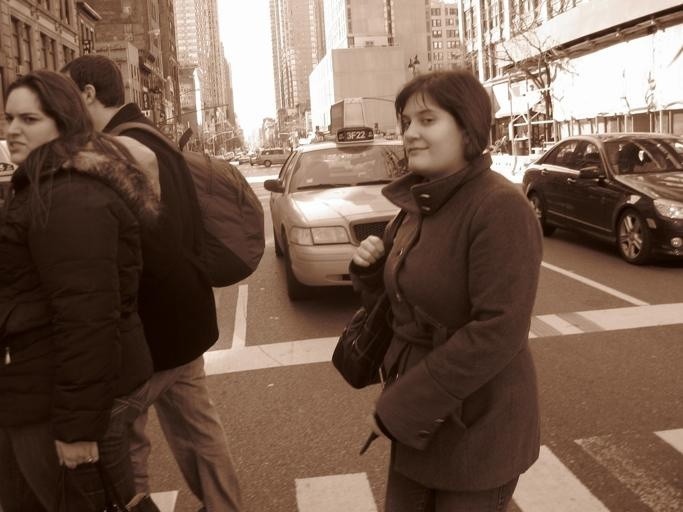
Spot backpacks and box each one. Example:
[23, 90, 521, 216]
[97, 119, 271, 290]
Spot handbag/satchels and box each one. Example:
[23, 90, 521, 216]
[51, 457, 164, 511]
[328, 288, 397, 393]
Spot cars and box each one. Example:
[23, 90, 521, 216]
[264, 126, 415, 301]
[521, 131, 683, 266]
[213, 145, 292, 168]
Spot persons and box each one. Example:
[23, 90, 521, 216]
[344, 66, 546, 512]
[58, 52, 244, 512]
[1, 66, 167, 512]
